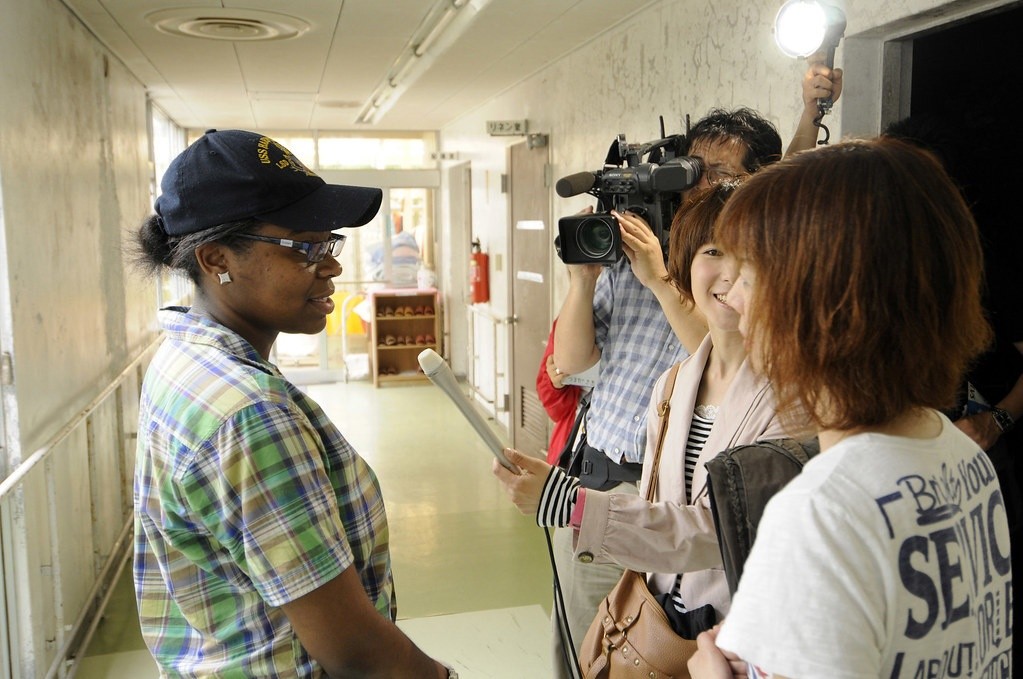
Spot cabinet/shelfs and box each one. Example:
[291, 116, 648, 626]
[369, 290, 443, 387]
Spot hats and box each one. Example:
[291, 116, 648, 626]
[155, 129, 384, 232]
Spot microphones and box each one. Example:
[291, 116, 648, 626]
[555, 170, 599, 198]
[417, 348, 522, 475]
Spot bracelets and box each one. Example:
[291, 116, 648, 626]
[443, 662, 459, 679]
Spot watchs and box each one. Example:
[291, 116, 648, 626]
[991, 407, 1015, 432]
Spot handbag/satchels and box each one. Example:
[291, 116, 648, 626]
[577, 567, 699, 678]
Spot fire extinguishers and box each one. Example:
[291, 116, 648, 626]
[468, 237, 491, 304]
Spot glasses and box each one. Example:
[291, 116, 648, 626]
[701, 167, 749, 186]
[230, 233, 346, 263]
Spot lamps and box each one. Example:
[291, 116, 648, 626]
[771, 0, 848, 106]
[355, 0, 489, 124]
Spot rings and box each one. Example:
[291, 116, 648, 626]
[555, 368, 560, 375]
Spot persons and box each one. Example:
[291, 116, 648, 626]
[488, 55, 1023, 679]
[129, 127, 460, 679]
